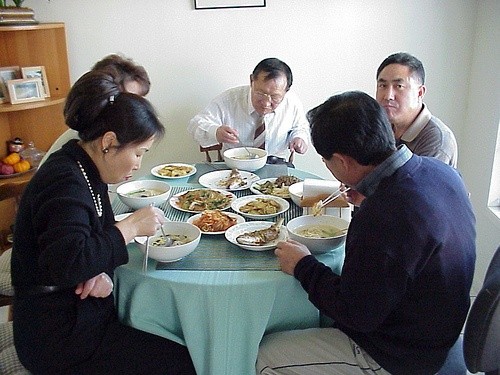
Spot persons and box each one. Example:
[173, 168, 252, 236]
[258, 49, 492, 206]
[255, 91, 478, 375]
[36, 54, 151, 172]
[10, 65, 198, 375]
[191, 58, 311, 168]
[375, 52, 458, 169]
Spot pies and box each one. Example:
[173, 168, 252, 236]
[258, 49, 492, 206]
[157, 165, 193, 177]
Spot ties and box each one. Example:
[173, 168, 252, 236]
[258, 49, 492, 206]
[254, 111, 265, 155]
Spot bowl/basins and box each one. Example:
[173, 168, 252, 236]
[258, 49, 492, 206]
[286, 212, 349, 254]
[223, 148, 268, 172]
[134, 221, 201, 262]
[288, 181, 303, 207]
[117, 179, 171, 210]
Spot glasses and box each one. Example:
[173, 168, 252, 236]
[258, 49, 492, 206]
[253, 77, 289, 102]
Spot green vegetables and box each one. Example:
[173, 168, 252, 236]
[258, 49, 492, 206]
[182, 191, 231, 209]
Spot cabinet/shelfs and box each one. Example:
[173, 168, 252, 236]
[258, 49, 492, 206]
[0, 21, 71, 241]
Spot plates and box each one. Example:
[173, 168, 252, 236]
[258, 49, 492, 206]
[198, 170, 260, 191]
[169, 188, 237, 213]
[230, 195, 290, 219]
[116, 213, 170, 244]
[250, 177, 290, 198]
[225, 221, 288, 251]
[187, 212, 245, 234]
[151, 163, 197, 179]
[0, 167, 35, 178]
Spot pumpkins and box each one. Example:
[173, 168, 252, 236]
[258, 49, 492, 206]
[0, 152, 30, 175]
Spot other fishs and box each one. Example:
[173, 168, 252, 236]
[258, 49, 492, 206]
[236, 218, 285, 246]
[226, 168, 242, 190]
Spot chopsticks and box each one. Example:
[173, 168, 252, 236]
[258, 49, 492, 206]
[141, 201, 155, 272]
[321, 184, 351, 208]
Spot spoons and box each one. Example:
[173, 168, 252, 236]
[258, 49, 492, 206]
[159, 225, 173, 247]
[234, 133, 260, 159]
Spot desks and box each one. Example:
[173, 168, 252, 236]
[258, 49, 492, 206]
[110, 162, 347, 375]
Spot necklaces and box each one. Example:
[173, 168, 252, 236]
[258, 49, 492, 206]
[78, 161, 103, 217]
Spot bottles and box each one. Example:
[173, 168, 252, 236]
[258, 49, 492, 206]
[20, 141, 44, 168]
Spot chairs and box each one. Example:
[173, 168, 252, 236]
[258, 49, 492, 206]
[0, 144, 500, 375]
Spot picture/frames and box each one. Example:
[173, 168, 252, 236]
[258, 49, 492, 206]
[0, 64, 51, 106]
